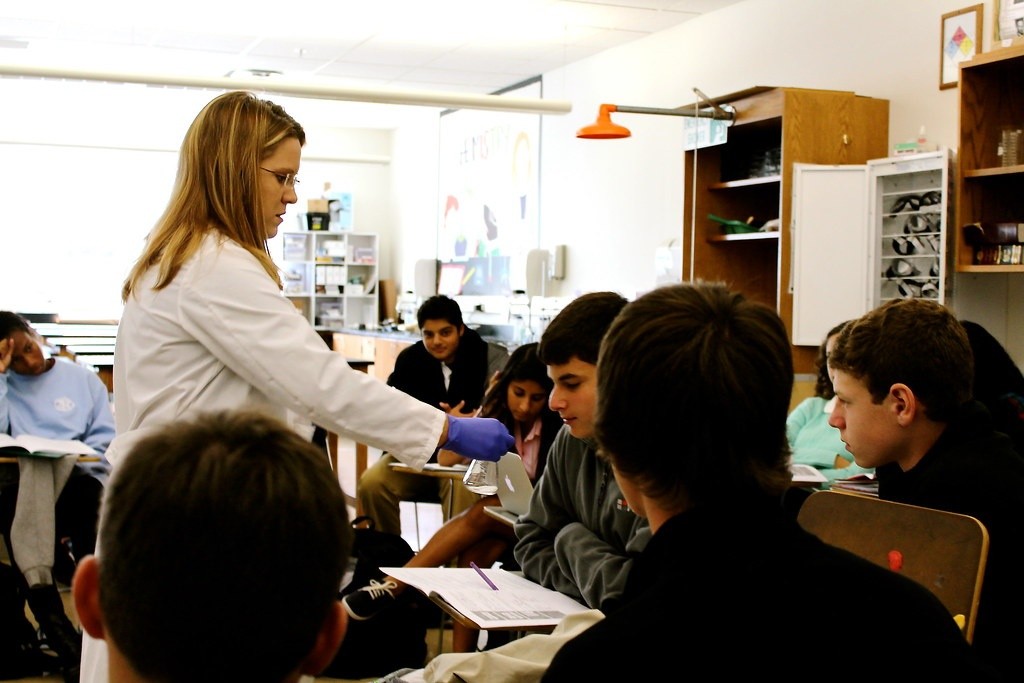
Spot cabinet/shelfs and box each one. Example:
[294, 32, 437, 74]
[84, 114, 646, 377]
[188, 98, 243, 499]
[791, 148, 955, 346]
[954, 44, 1024, 272]
[681, 87, 890, 374]
[333, 333, 416, 385]
[280, 231, 380, 327]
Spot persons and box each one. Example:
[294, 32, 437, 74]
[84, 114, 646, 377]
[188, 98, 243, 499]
[360, 296, 509, 537]
[512, 293, 652, 616]
[787, 319, 876, 520]
[79, 92, 517, 683]
[541, 281, 1002, 683]
[827, 298, 1024, 683]
[0, 309, 116, 683]
[72, 407, 347, 683]
[342, 343, 564, 654]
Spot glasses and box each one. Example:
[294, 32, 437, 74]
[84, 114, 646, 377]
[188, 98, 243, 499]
[258, 164, 300, 189]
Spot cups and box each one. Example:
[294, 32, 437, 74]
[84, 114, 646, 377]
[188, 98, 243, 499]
[750, 149, 781, 177]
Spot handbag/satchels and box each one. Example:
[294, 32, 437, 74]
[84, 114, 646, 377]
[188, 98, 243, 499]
[317, 511, 431, 680]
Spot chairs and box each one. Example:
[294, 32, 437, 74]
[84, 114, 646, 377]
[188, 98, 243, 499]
[797, 490, 991, 644]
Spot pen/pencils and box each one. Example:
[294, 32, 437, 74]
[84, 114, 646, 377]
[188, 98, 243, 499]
[470, 561, 499, 591]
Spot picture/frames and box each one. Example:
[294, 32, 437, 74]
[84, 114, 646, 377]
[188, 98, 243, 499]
[939, 2, 984, 90]
[991, 0, 1024, 51]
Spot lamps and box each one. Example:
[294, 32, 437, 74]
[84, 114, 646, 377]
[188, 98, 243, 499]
[576, 103, 738, 140]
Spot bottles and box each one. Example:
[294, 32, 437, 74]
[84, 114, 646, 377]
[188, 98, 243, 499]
[463, 459, 498, 495]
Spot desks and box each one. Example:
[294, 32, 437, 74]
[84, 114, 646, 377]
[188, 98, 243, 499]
[0, 446, 590, 654]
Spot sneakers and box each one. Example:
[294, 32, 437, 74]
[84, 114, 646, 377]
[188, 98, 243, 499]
[342, 575, 397, 621]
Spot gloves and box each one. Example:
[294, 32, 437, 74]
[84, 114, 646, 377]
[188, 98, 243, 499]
[443, 418, 517, 462]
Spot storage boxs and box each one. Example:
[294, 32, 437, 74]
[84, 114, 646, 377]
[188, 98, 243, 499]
[307, 198, 330, 214]
